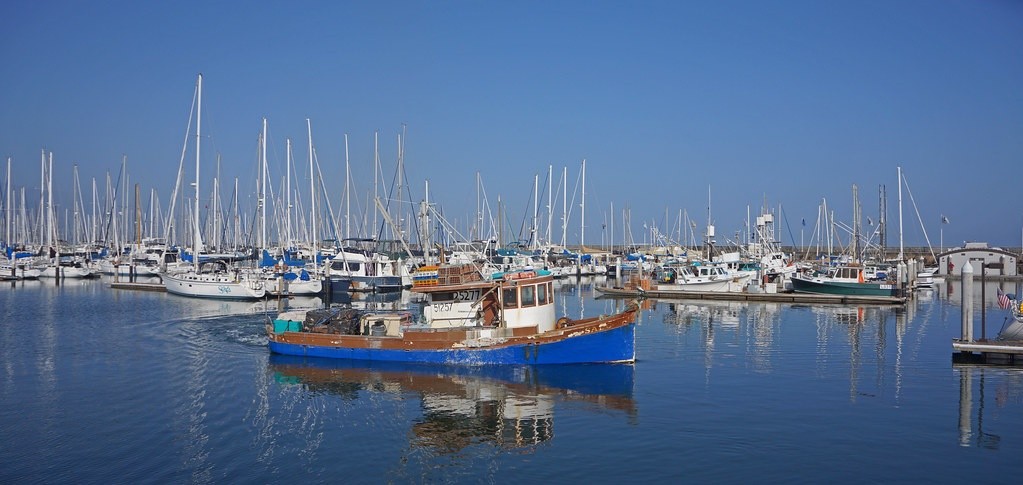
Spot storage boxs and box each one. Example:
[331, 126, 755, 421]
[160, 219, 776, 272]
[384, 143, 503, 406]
[414, 263, 481, 287]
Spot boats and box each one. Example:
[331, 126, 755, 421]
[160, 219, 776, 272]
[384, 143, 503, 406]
[262, 260, 640, 366]
[266, 352, 637, 457]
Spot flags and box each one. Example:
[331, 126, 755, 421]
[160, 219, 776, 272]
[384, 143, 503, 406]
[602, 224, 606, 229]
[997, 286, 1011, 309]
[802, 219, 805, 224]
[868, 217, 873, 226]
[942, 215, 950, 224]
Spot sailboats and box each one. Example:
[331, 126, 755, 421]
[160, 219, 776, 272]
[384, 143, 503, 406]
[0, 71, 940, 306]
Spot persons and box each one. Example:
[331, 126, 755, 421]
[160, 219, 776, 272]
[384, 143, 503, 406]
[949, 262, 954, 275]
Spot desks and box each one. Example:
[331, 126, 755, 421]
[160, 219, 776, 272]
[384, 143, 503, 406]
[367, 314, 412, 337]
